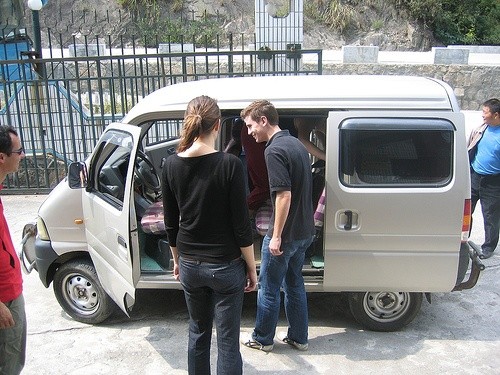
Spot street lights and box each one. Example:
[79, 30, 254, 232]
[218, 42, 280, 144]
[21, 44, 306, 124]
[28, 0, 43, 79]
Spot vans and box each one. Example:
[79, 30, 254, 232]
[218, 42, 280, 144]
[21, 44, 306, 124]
[16, 74, 485, 332]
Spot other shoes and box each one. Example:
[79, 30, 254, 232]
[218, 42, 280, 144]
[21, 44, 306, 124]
[242, 336, 274, 352]
[480, 245, 495, 258]
[277, 331, 308, 350]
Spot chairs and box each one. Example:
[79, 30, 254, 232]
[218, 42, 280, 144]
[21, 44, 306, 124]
[255, 185, 326, 257]
[141, 202, 179, 233]
[224, 119, 242, 155]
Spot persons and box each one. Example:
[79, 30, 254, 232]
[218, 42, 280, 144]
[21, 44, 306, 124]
[0, 125, 26, 375]
[239, 99, 316, 351]
[465, 98, 500, 260]
[222, 114, 325, 210]
[161, 95, 259, 374]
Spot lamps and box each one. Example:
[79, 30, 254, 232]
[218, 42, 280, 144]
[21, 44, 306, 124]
[27, 0, 42, 10]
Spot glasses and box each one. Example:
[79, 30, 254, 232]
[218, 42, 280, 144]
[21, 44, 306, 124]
[2, 146, 23, 155]
